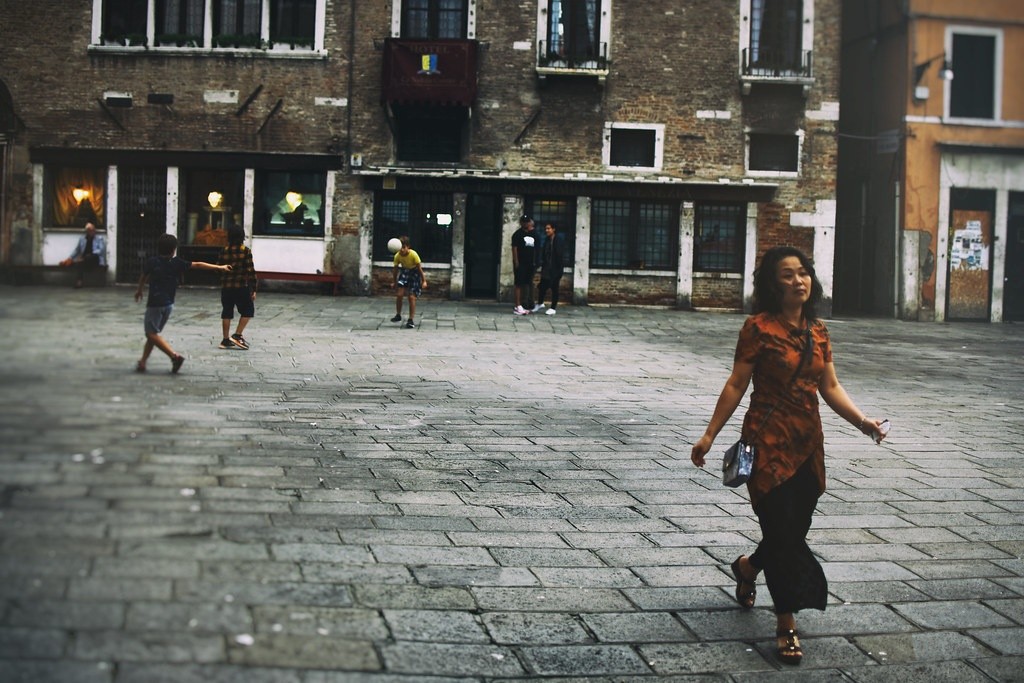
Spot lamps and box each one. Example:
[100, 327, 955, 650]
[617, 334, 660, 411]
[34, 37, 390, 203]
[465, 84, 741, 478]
[913, 52, 955, 84]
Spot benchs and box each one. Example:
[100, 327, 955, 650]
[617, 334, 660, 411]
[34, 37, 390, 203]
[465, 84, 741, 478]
[255, 271, 342, 296]
[7, 265, 107, 286]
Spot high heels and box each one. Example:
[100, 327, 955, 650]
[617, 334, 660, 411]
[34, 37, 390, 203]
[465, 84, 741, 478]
[777, 629, 802, 664]
[731, 555, 756, 607]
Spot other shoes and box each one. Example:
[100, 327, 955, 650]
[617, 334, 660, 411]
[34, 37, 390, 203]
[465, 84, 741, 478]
[75, 279, 83, 288]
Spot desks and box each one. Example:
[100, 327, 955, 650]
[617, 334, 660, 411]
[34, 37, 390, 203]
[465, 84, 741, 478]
[178, 246, 224, 286]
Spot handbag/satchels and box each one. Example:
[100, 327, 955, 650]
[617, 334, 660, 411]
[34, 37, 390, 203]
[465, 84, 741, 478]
[722, 440, 754, 488]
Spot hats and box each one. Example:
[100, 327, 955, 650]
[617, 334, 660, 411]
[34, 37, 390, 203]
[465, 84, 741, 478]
[520, 215, 530, 222]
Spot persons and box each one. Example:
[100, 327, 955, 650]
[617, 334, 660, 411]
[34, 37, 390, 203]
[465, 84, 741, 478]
[66, 224, 109, 289]
[511, 214, 566, 316]
[133, 234, 234, 374]
[691, 245, 890, 663]
[390, 237, 428, 328]
[217, 225, 259, 350]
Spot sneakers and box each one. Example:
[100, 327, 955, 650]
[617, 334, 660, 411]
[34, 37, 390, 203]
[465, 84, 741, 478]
[391, 314, 401, 321]
[229, 334, 249, 349]
[406, 319, 414, 328]
[545, 308, 556, 315]
[172, 355, 185, 373]
[514, 305, 529, 315]
[135, 364, 145, 372]
[219, 338, 235, 348]
[531, 303, 545, 313]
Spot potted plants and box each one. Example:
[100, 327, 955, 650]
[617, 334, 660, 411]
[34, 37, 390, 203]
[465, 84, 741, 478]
[101, 32, 313, 50]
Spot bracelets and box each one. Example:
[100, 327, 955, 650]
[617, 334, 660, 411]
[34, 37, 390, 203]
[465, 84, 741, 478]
[859, 416, 865, 432]
